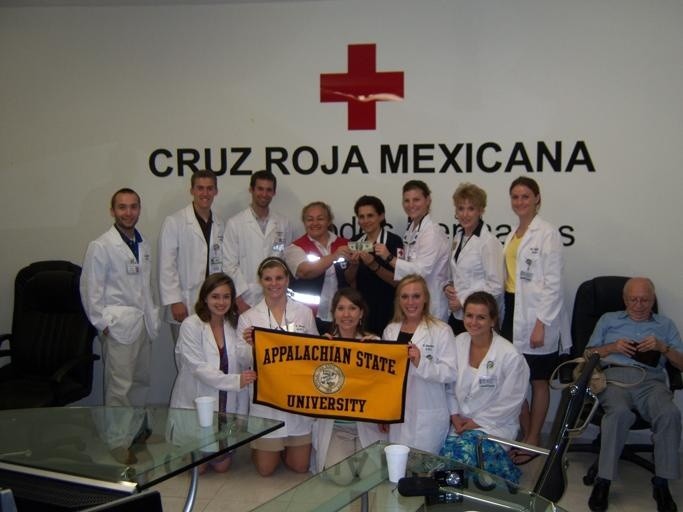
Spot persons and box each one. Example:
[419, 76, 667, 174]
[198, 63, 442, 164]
[382, 275, 459, 455]
[308, 285, 388, 483]
[440, 290, 531, 485]
[284, 201, 353, 335]
[236, 256, 321, 475]
[351, 196, 405, 336]
[502, 177, 573, 464]
[157, 171, 226, 344]
[223, 171, 295, 312]
[168, 272, 256, 474]
[394, 179, 453, 323]
[80, 188, 163, 466]
[442, 182, 507, 337]
[585, 277, 683, 512]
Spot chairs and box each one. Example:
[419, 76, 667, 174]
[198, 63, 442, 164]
[561, 275, 683, 487]
[0, 260, 99, 409]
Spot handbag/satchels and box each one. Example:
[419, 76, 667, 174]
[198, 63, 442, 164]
[550, 357, 645, 391]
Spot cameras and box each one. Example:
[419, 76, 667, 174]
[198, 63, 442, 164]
[433, 467, 463, 487]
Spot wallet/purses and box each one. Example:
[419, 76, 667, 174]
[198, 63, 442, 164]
[628, 339, 660, 368]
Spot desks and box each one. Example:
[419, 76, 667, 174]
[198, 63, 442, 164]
[246, 440, 568, 512]
[0, 404, 284, 489]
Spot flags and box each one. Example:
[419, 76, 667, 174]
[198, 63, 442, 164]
[247, 325, 412, 424]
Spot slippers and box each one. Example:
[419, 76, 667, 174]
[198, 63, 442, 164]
[508, 443, 519, 457]
[512, 445, 542, 464]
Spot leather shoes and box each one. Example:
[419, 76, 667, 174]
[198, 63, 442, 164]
[113, 447, 137, 464]
[588, 479, 611, 512]
[653, 479, 678, 511]
[135, 432, 161, 444]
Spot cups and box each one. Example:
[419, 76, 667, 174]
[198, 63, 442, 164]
[384, 445, 410, 483]
[194, 396, 215, 428]
